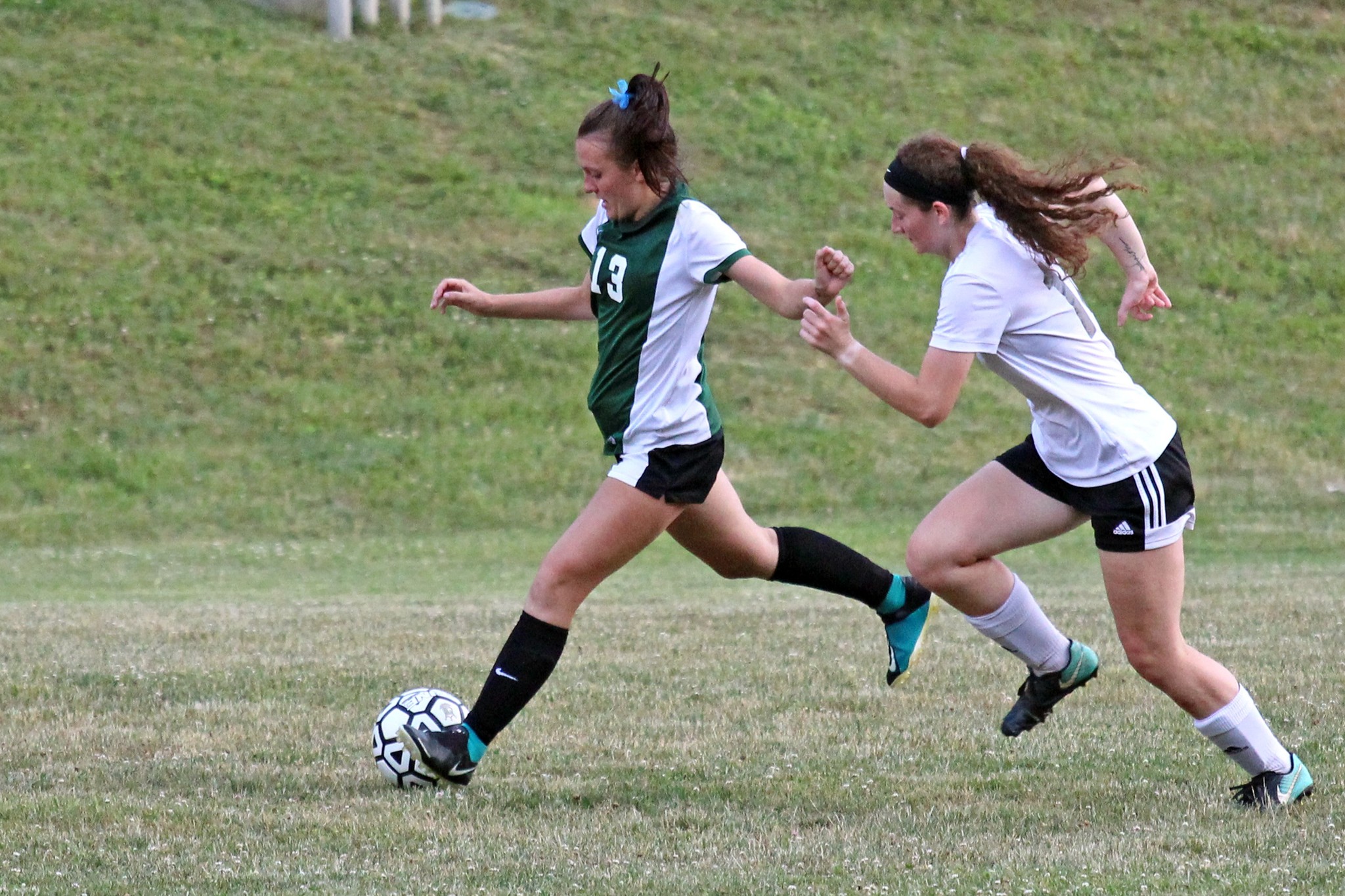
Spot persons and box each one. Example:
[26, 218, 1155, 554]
[396, 74, 931, 788]
[797, 140, 1315, 808]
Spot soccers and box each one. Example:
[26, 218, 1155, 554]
[370, 687, 471, 790]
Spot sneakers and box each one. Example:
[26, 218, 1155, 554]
[879, 571, 938, 690]
[1230, 752, 1315, 811]
[1001, 635, 1101, 738]
[395, 723, 478, 789]
